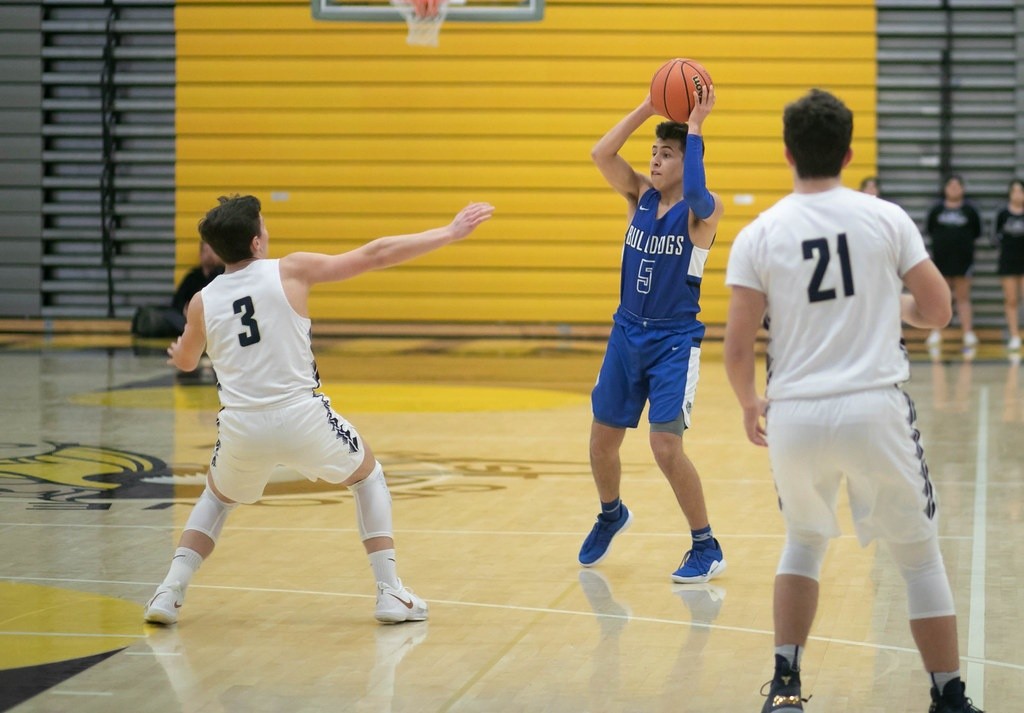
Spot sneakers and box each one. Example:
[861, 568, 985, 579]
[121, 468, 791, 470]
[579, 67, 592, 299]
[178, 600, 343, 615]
[576, 503, 634, 569]
[143, 585, 184, 626]
[758, 679, 815, 713]
[927, 681, 983, 713]
[925, 332, 944, 345]
[373, 578, 430, 625]
[1007, 337, 1021, 351]
[963, 332, 979, 347]
[670, 540, 728, 584]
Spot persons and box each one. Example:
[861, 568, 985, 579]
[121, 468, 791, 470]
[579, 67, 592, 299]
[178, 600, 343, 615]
[142, 196, 498, 627]
[578, 83, 728, 586]
[859, 170, 985, 348]
[992, 179, 1024, 349]
[724, 87, 976, 713]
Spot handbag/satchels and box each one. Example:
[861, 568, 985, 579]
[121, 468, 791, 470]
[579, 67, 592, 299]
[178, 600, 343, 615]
[131, 303, 186, 338]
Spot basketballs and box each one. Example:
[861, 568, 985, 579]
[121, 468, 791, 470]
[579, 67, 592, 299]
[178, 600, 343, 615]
[650, 56, 714, 123]
